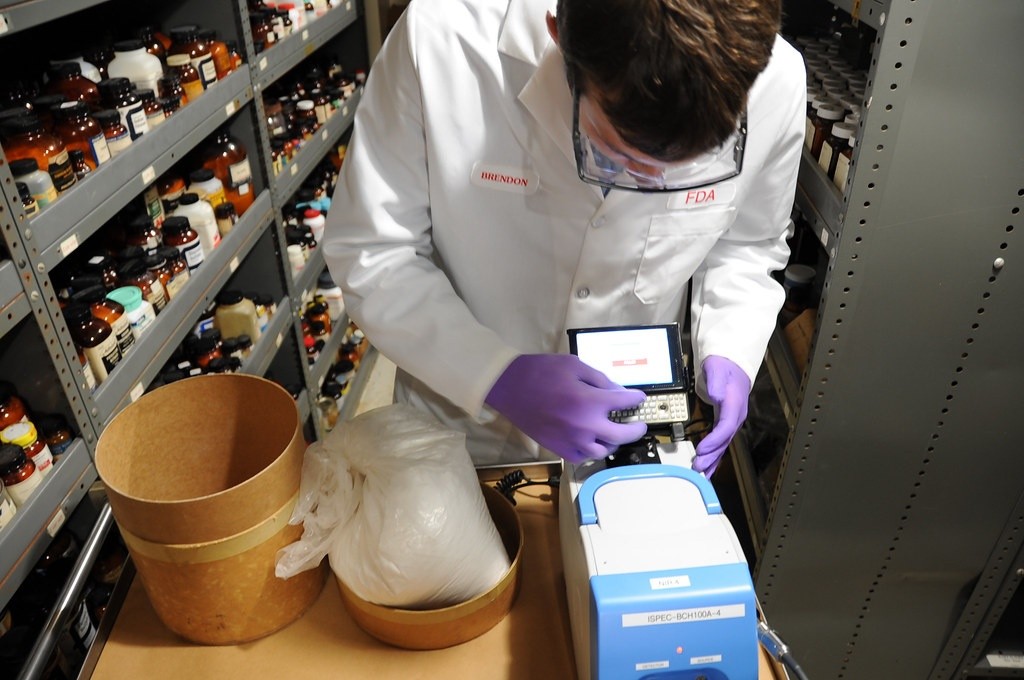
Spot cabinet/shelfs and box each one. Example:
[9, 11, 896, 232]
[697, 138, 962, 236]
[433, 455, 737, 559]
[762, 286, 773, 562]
[0, 0, 378, 680]
[682, 0, 1024, 680]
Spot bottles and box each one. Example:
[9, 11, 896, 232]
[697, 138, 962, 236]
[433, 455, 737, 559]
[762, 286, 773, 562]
[0, 22, 239, 221]
[0, 525, 131, 680]
[48, 125, 285, 396]
[299, 270, 345, 371]
[282, 145, 347, 281]
[0, 379, 78, 532]
[246, 0, 342, 55]
[265, 58, 367, 178]
[315, 321, 368, 433]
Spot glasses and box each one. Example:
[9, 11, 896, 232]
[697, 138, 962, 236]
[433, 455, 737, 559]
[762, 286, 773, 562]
[572, 80, 748, 193]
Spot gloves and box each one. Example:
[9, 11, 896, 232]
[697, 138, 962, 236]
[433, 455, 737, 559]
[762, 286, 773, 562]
[485, 353, 647, 464]
[692, 355, 751, 480]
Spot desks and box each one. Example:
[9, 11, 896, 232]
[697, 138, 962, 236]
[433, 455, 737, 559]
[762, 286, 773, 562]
[90, 464, 776, 680]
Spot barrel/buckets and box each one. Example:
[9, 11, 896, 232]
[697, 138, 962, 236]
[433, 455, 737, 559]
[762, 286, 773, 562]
[94, 372, 330, 646]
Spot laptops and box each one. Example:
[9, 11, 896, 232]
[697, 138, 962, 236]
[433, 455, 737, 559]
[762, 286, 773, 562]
[565, 322, 689, 426]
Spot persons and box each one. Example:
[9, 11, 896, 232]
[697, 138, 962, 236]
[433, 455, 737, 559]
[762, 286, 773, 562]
[320, 0, 807, 479]
